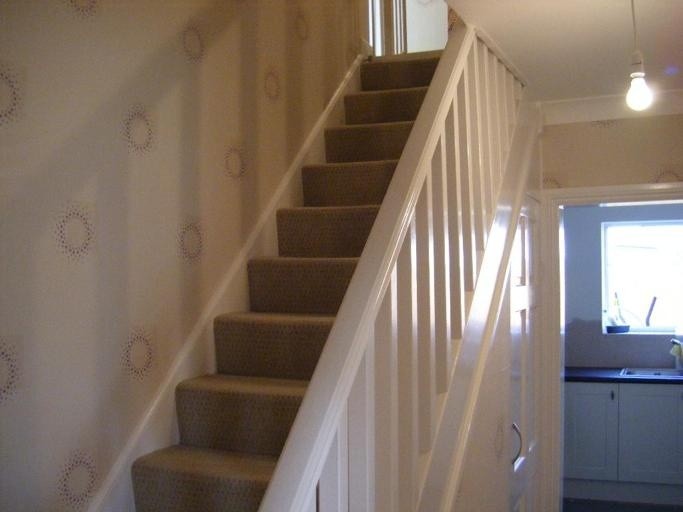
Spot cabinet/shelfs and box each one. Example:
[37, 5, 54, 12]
[564, 382, 683, 505]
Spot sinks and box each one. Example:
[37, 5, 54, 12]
[620, 367, 683, 378]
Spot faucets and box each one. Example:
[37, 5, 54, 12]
[670, 338, 683, 347]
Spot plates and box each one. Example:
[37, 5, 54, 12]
[606, 325, 629, 333]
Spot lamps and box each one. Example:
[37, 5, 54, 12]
[625, 0, 655, 112]
